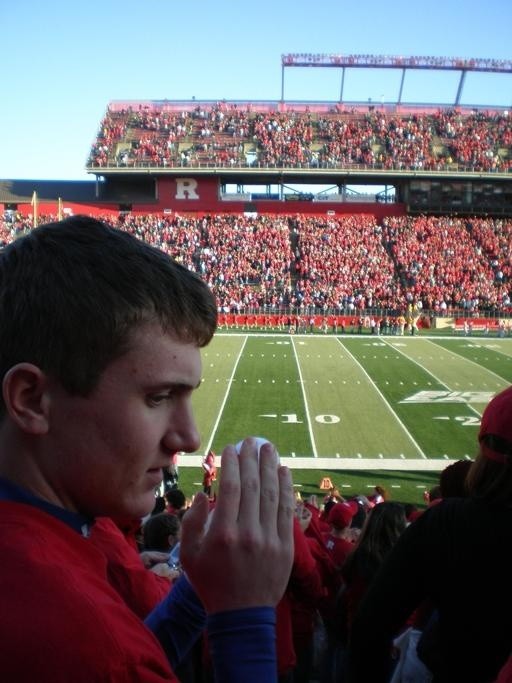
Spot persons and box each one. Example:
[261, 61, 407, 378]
[2, 211, 300, 682]
[338, 382, 511, 681]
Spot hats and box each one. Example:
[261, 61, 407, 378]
[477, 385, 511, 464]
[328, 500, 358, 528]
